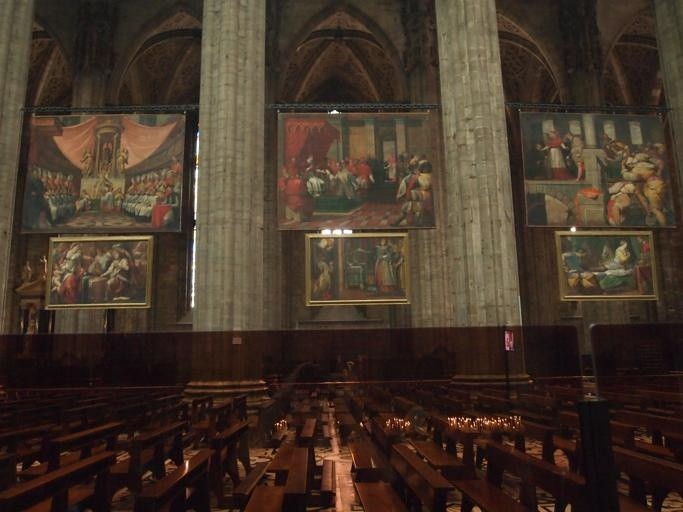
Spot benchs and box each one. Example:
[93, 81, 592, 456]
[1, 373, 683, 512]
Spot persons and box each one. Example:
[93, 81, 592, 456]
[560, 238, 598, 294]
[26, 141, 182, 226]
[50, 241, 148, 303]
[280, 150, 436, 227]
[315, 253, 333, 300]
[612, 239, 633, 270]
[526, 115, 672, 225]
[373, 238, 397, 294]
[392, 240, 405, 297]
[631, 235, 654, 292]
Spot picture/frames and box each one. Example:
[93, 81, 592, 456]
[44, 237, 154, 311]
[305, 232, 412, 307]
[554, 231, 659, 302]
